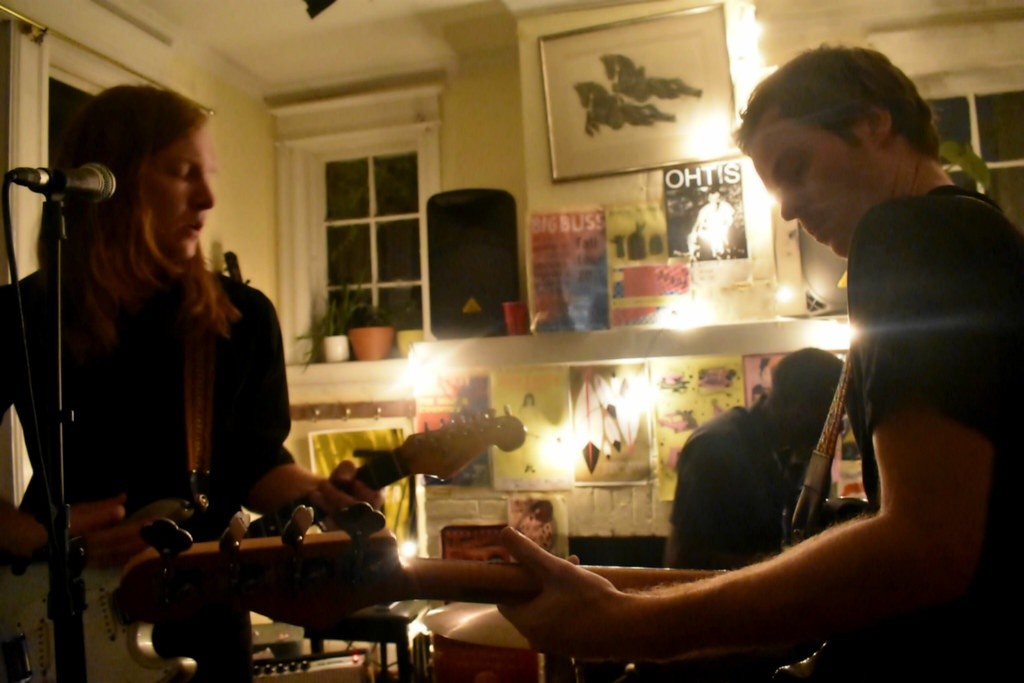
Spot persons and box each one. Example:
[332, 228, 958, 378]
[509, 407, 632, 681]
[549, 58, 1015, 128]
[684, 189, 736, 259]
[0, 85, 387, 683]
[498, 45, 1024, 683]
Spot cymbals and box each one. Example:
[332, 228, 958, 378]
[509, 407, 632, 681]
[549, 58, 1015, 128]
[422, 600, 534, 650]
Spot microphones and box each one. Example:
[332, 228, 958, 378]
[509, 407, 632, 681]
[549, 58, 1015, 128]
[12, 163, 117, 203]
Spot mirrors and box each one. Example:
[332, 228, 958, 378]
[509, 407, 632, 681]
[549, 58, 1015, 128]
[308, 423, 427, 559]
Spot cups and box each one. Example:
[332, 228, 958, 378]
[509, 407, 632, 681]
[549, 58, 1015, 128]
[504, 302, 530, 334]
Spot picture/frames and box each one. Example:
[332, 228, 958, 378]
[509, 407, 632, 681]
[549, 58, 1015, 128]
[537, 3, 743, 186]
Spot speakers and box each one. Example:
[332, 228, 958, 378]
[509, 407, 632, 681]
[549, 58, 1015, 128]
[426, 188, 521, 340]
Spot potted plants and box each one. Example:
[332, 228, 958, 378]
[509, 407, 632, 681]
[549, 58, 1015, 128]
[298, 272, 365, 373]
[347, 306, 396, 360]
[394, 301, 423, 357]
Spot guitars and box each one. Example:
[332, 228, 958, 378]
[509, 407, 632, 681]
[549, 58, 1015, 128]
[114, 497, 862, 683]
[0, 406, 526, 683]
